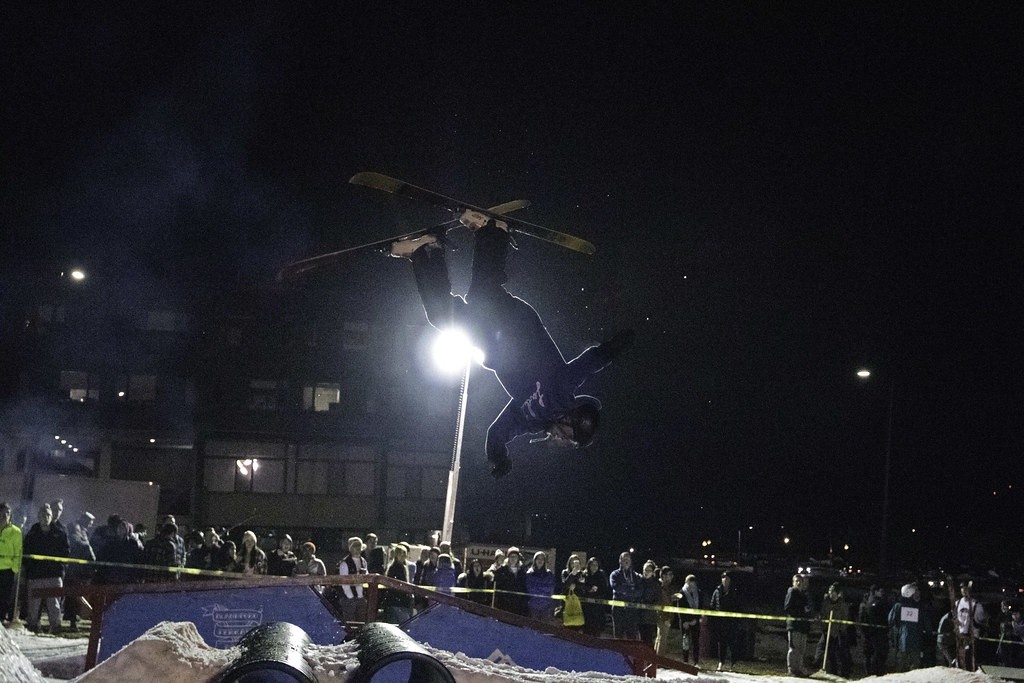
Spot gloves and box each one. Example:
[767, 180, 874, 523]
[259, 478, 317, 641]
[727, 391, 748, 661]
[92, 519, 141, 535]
[598, 329, 635, 359]
[490, 455, 513, 480]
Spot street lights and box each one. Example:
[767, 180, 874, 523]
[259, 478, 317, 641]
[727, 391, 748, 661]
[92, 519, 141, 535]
[21, 266, 84, 514]
[738, 525, 753, 557]
[857, 366, 895, 571]
[441, 331, 473, 546]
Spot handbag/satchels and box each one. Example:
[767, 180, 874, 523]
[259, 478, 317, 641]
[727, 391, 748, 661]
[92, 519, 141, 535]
[563, 589, 584, 626]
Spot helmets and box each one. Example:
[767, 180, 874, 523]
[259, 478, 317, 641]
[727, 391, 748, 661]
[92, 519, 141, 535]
[571, 395, 601, 449]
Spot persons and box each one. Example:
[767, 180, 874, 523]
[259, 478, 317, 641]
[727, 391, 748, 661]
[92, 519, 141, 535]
[459, 545, 608, 637]
[185, 528, 326, 576]
[0, 497, 184, 635]
[783, 573, 1024, 678]
[610, 551, 772, 671]
[340, 533, 462, 622]
[380, 208, 637, 479]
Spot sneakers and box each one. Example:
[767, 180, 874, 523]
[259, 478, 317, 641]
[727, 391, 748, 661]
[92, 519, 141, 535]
[390, 235, 444, 259]
[460, 212, 508, 232]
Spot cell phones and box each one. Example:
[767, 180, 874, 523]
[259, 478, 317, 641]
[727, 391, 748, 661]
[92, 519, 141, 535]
[574, 560, 580, 572]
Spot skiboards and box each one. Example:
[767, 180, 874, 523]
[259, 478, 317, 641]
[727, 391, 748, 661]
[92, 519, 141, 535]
[945, 574, 976, 672]
[286, 172, 597, 274]
